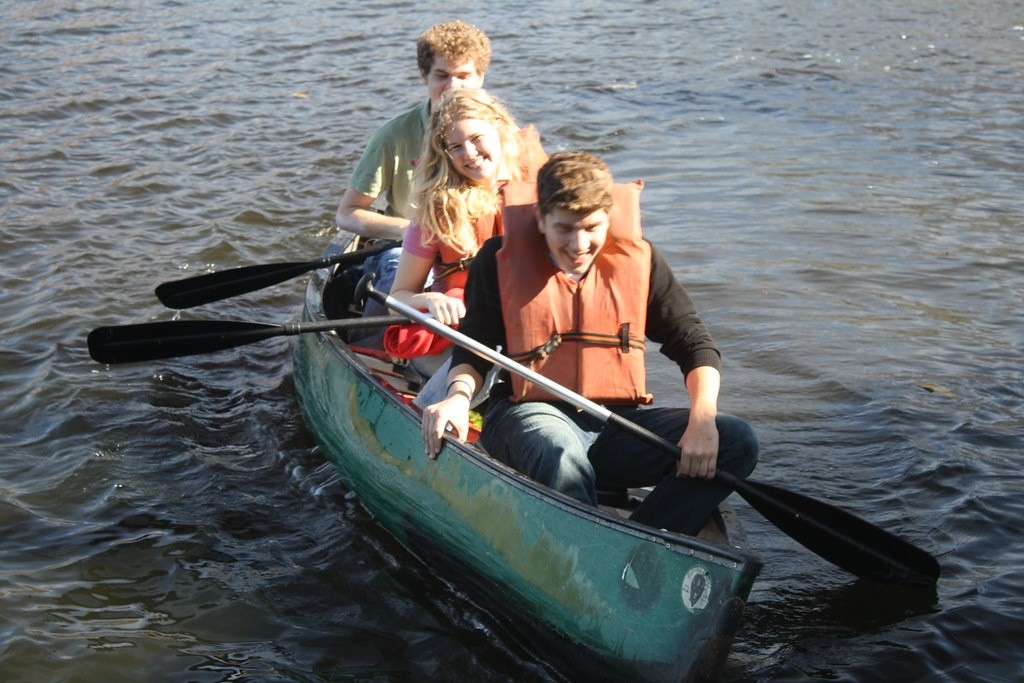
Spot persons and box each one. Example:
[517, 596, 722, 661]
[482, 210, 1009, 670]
[335, 23, 489, 340]
[421, 153, 760, 535]
[386, 89, 540, 408]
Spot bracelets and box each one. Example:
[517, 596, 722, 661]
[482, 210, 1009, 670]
[446, 380, 472, 396]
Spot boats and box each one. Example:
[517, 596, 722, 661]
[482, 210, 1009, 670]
[292, 190, 766, 683]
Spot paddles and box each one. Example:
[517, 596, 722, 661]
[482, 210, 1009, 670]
[153, 241, 404, 311]
[86, 311, 433, 366]
[354, 271, 944, 585]
